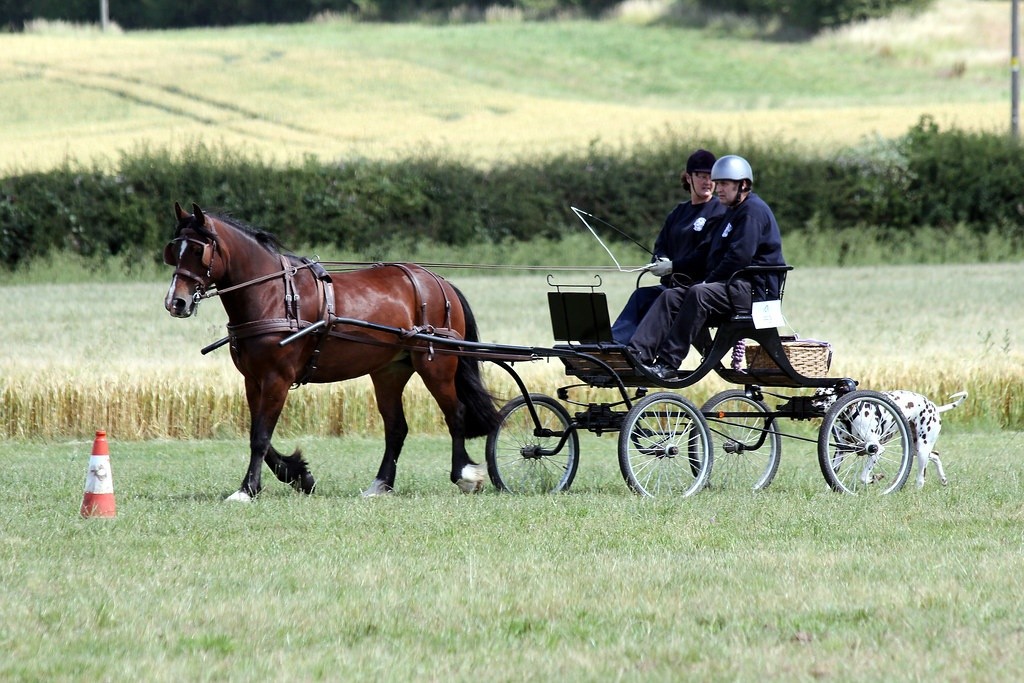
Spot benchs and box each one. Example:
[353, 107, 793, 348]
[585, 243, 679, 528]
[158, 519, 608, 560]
[610, 262, 792, 378]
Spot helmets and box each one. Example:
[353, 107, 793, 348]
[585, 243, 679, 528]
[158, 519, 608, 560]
[687, 149, 716, 174]
[711, 154, 753, 185]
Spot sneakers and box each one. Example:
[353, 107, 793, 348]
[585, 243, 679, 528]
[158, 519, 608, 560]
[641, 359, 678, 382]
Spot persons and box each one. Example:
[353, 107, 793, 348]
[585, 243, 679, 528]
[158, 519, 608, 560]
[628, 155, 786, 380]
[611, 149, 728, 348]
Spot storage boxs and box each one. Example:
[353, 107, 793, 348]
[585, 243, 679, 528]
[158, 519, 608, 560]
[743, 341, 829, 378]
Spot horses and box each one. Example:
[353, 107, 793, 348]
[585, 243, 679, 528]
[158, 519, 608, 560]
[162, 202, 512, 505]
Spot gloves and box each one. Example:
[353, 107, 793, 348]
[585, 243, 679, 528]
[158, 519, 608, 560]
[649, 257, 673, 276]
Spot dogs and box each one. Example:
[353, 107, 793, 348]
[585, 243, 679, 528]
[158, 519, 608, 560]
[805, 388, 968, 493]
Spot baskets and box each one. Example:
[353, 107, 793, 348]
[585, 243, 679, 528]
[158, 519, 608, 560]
[746, 344, 828, 378]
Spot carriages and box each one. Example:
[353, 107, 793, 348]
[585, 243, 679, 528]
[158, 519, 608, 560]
[162, 198, 915, 509]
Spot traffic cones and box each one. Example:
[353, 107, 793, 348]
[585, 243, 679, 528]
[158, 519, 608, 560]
[75, 430, 118, 518]
